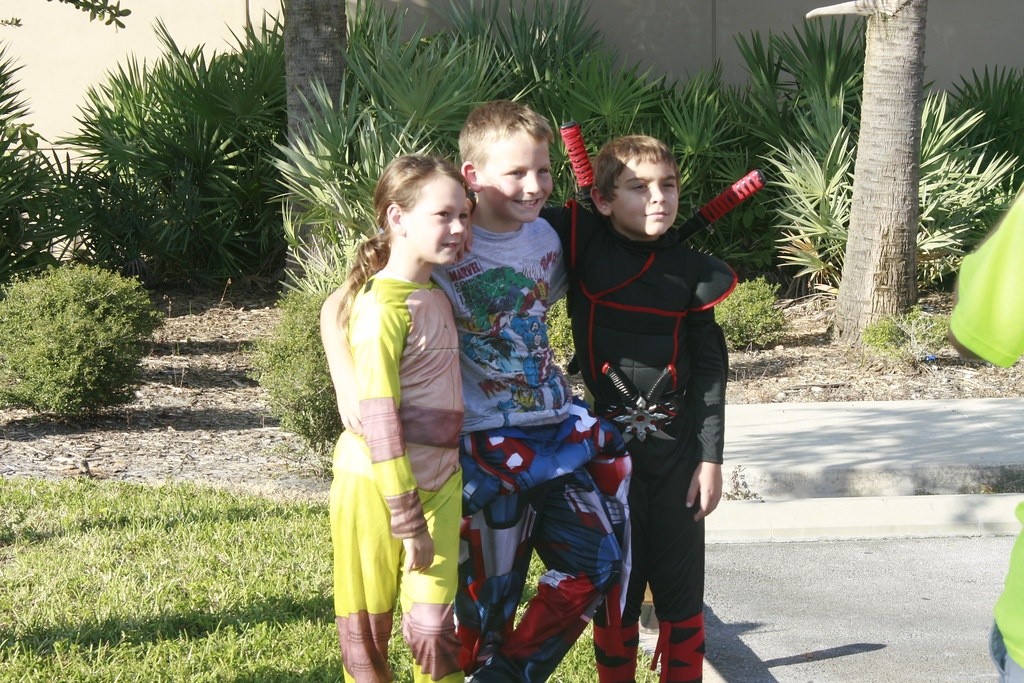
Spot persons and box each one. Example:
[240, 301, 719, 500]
[451, 135, 738, 683]
[946, 191, 1024, 683]
[327, 154, 470, 683]
[317, 100, 636, 683]
